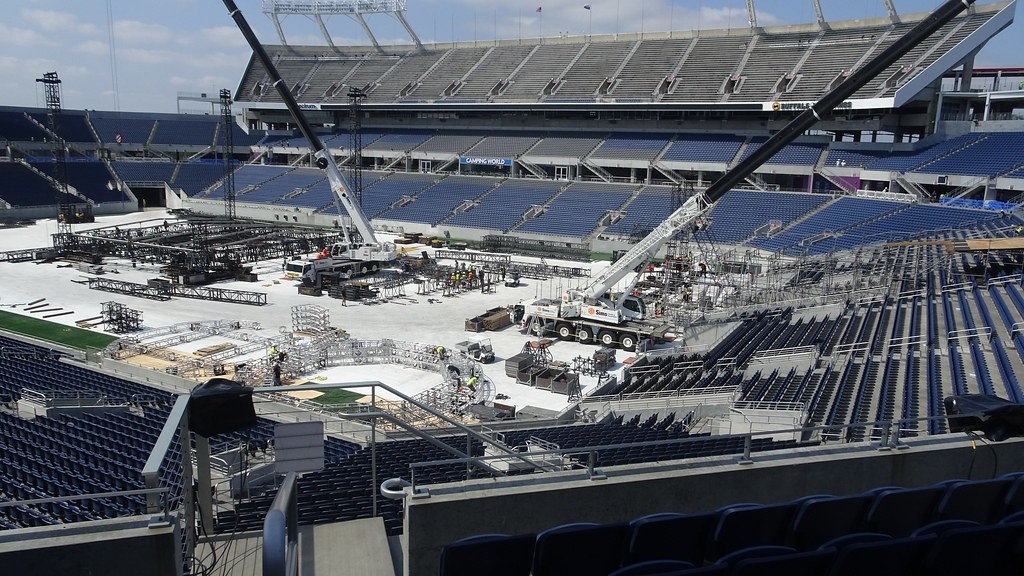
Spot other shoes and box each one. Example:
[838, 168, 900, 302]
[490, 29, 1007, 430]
[472, 396, 475, 400]
[468, 395, 471, 400]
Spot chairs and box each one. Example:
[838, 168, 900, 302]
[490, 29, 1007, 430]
[0, 106, 1024, 576]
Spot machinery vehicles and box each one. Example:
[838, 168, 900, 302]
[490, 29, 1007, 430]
[510, 0, 977, 352]
[504, 268, 520, 287]
[224, 0, 397, 280]
[57, 207, 69, 223]
[76, 208, 94, 223]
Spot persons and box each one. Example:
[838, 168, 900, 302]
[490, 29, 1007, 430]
[654, 300, 665, 316]
[432, 346, 481, 393]
[317, 246, 331, 259]
[835, 158, 847, 167]
[269, 344, 288, 387]
[682, 281, 691, 303]
[699, 263, 708, 279]
[341, 286, 347, 307]
[448, 260, 484, 288]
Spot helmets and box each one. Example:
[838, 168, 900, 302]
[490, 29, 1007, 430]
[475, 374, 479, 378]
[272, 344, 277, 347]
